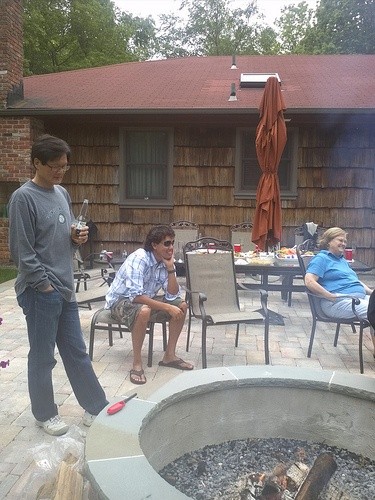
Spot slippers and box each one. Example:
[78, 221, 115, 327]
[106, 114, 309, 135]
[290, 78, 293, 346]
[130, 369, 146, 384]
[159, 358, 193, 370]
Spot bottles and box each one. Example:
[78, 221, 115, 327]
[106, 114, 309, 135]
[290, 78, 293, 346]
[72, 199, 89, 246]
[100, 244, 104, 260]
[122, 243, 127, 261]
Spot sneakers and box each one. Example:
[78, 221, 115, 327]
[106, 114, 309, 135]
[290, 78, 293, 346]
[82, 410, 97, 426]
[35, 415, 69, 435]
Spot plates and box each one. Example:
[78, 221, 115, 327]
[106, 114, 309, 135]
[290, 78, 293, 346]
[276, 255, 298, 261]
[301, 254, 316, 257]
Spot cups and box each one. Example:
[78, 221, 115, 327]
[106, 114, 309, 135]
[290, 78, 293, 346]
[234, 243, 241, 253]
[345, 249, 352, 260]
[106, 252, 113, 261]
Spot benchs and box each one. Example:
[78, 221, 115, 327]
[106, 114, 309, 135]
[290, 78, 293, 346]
[93, 258, 126, 283]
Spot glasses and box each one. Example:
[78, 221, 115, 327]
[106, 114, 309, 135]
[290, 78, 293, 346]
[160, 240, 175, 247]
[46, 163, 69, 172]
[333, 238, 347, 244]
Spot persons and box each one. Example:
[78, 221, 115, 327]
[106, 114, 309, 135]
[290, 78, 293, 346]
[105, 226, 194, 385]
[305, 227, 375, 359]
[7, 134, 108, 436]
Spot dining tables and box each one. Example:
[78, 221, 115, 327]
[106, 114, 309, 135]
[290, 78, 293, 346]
[174, 253, 372, 301]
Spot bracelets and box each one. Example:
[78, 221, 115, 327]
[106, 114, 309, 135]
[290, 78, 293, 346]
[168, 269, 177, 273]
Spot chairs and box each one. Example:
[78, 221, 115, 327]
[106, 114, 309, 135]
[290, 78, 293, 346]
[230, 222, 262, 283]
[297, 238, 370, 374]
[89, 308, 167, 367]
[73, 248, 117, 310]
[169, 219, 202, 254]
[288, 224, 325, 306]
[183, 237, 269, 369]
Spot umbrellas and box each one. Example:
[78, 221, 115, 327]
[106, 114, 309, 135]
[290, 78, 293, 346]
[251, 77, 288, 290]
